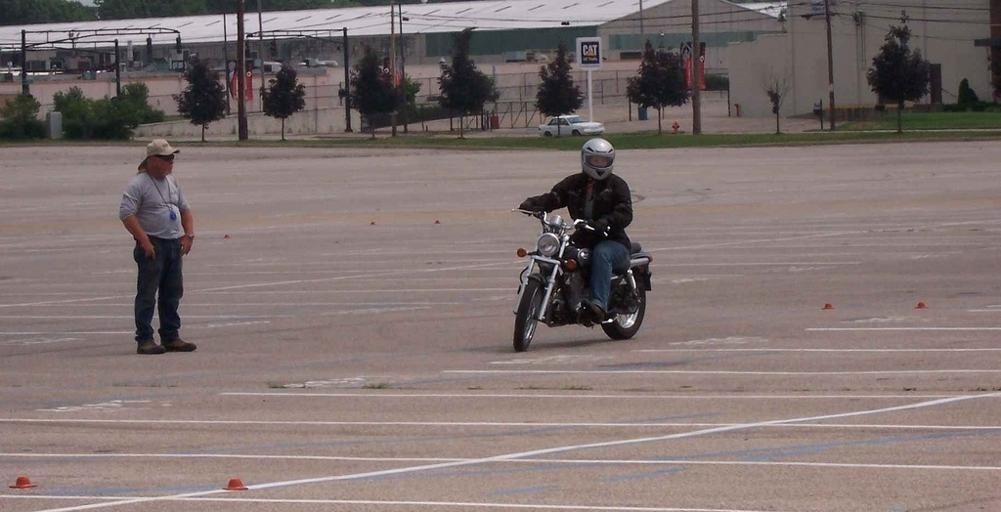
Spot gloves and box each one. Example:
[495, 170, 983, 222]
[593, 218, 610, 236]
[519, 198, 536, 217]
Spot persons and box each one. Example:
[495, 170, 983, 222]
[519, 138, 632, 325]
[119, 139, 198, 355]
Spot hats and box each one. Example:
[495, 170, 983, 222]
[145, 138, 180, 158]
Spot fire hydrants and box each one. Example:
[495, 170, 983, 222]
[672, 121, 680, 132]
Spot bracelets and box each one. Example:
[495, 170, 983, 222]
[187, 233, 194, 239]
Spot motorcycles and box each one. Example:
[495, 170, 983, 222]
[511, 208, 653, 352]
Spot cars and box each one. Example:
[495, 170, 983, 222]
[538, 115, 605, 137]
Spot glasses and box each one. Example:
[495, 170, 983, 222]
[158, 155, 176, 161]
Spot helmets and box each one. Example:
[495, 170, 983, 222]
[580, 137, 615, 181]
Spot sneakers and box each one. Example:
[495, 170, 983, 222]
[136, 340, 167, 354]
[161, 339, 197, 352]
[581, 298, 605, 325]
